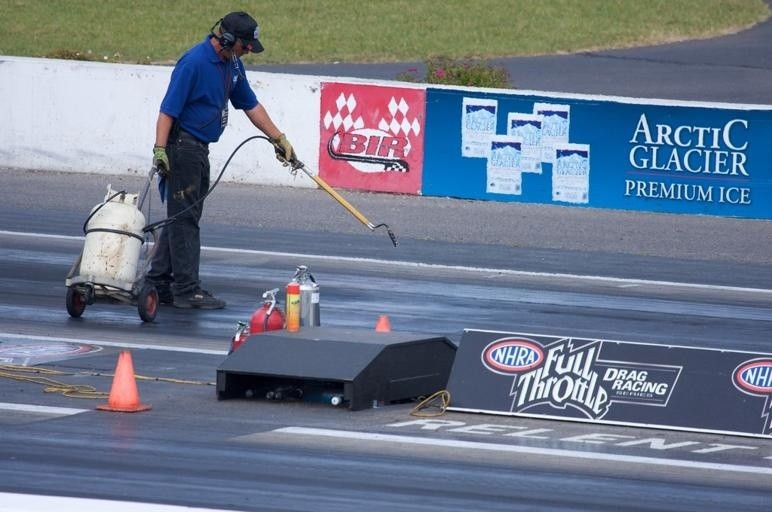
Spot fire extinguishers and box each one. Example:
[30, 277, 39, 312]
[232, 265, 320, 355]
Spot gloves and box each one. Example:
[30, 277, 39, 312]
[153, 147, 170, 176]
[274, 133, 298, 166]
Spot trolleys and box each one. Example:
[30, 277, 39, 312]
[66, 159, 162, 324]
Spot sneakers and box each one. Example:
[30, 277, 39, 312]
[144, 279, 225, 310]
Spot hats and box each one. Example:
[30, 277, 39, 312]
[221, 11, 264, 53]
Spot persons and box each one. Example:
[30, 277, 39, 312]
[145, 11, 297, 309]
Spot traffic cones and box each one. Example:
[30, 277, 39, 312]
[97, 345, 154, 417]
